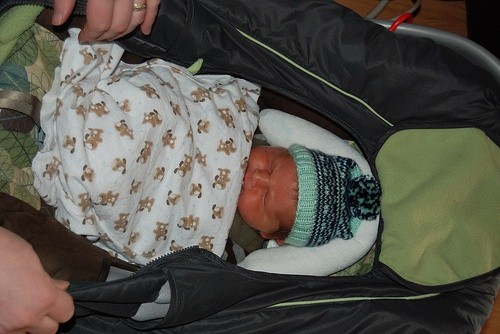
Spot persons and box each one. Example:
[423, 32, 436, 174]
[63, 75, 349, 262]
[0, 0, 164, 334]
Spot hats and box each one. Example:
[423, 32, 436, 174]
[284, 143, 383, 248]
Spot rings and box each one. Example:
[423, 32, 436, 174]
[131, 2, 147, 15]
